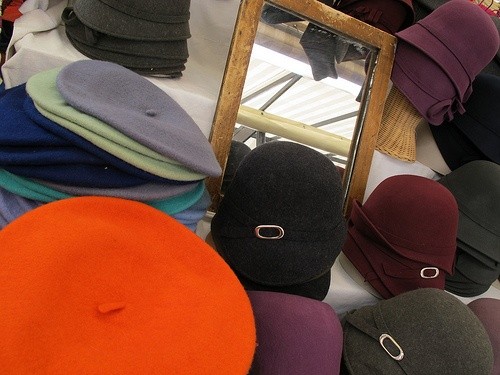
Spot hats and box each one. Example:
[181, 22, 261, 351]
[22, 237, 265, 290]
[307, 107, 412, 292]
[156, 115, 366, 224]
[0, 0, 500, 375]
[222, 141, 252, 194]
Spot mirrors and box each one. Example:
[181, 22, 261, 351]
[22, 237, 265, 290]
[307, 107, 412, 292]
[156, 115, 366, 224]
[211, 0, 399, 226]
